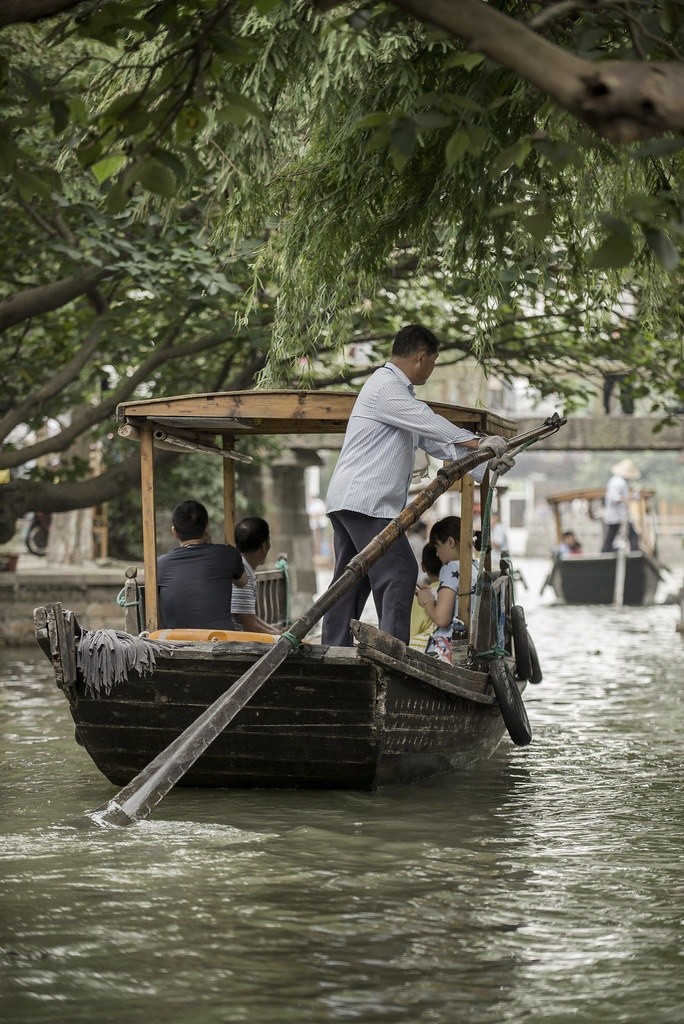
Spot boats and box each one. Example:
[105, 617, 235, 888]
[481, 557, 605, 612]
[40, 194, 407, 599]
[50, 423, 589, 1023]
[546, 488, 661, 608]
[28, 388, 544, 798]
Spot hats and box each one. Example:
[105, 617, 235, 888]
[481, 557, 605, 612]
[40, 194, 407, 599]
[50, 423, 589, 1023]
[611, 458, 641, 480]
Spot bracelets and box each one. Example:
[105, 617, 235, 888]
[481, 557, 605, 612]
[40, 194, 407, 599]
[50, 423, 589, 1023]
[421, 599, 435, 608]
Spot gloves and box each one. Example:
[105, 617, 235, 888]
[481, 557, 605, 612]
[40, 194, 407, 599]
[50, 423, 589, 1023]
[477, 435, 509, 460]
[487, 453, 515, 476]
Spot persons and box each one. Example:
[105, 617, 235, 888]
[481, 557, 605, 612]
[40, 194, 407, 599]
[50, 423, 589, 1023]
[599, 458, 641, 553]
[230, 518, 283, 633]
[156, 498, 247, 629]
[564, 533, 582, 554]
[321, 325, 516, 664]
